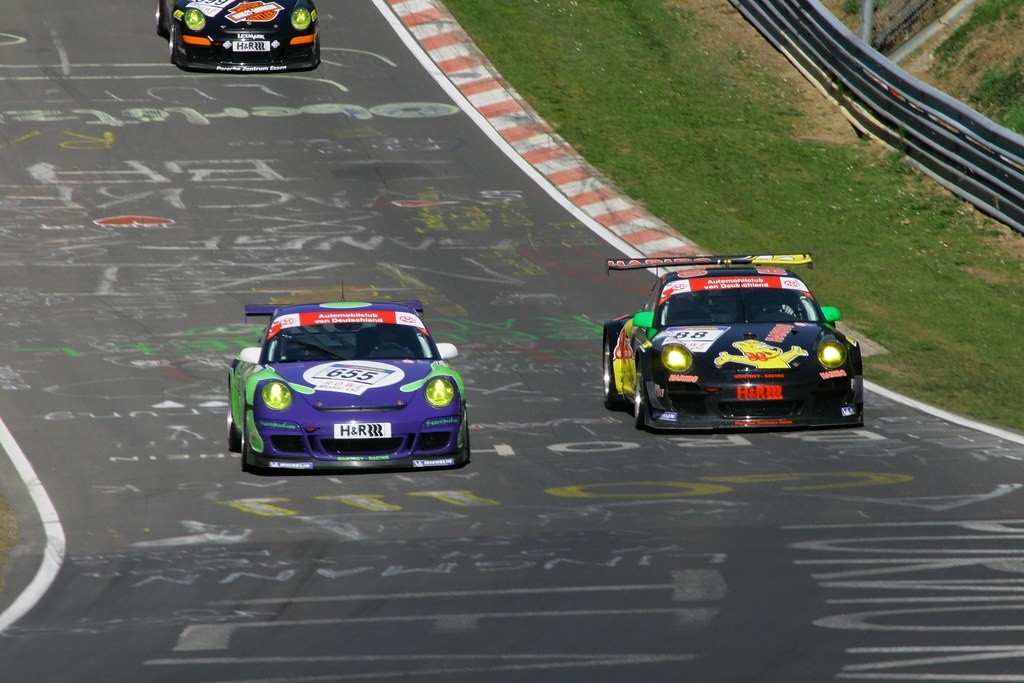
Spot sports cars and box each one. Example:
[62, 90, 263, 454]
[222, 300, 469, 473]
[598, 253, 864, 434]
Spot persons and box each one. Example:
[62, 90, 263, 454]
[350, 323, 417, 358]
[759, 290, 797, 320]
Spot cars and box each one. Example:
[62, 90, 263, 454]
[151, 0, 320, 72]
